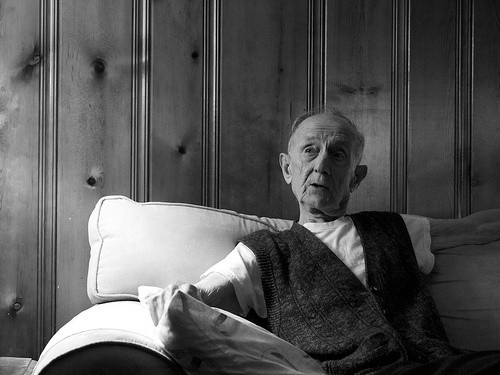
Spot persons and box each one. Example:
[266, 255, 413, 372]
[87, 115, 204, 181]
[149, 108, 500, 375]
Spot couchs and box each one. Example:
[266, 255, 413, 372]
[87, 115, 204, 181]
[31, 195, 500, 375]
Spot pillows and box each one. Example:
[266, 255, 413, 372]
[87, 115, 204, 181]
[137, 286, 327, 375]
